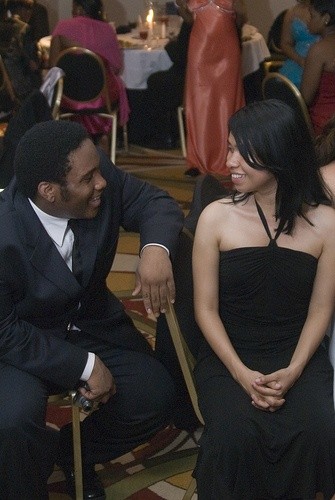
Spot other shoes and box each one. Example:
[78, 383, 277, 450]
[187, 167, 198, 175]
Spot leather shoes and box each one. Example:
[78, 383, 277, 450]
[170, 113, 186, 130]
[60, 422, 106, 500]
[136, 126, 175, 148]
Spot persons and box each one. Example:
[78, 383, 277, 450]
[0, 118, 187, 500]
[191, 98, 335, 500]
[0, 0, 335, 207]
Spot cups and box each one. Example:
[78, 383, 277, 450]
[138, 15, 150, 38]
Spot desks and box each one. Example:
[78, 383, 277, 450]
[37, 20, 272, 145]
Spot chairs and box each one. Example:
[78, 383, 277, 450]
[0, 45, 335, 500]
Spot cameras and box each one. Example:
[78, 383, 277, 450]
[73, 384, 94, 412]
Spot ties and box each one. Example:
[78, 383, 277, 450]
[68, 218, 81, 285]
